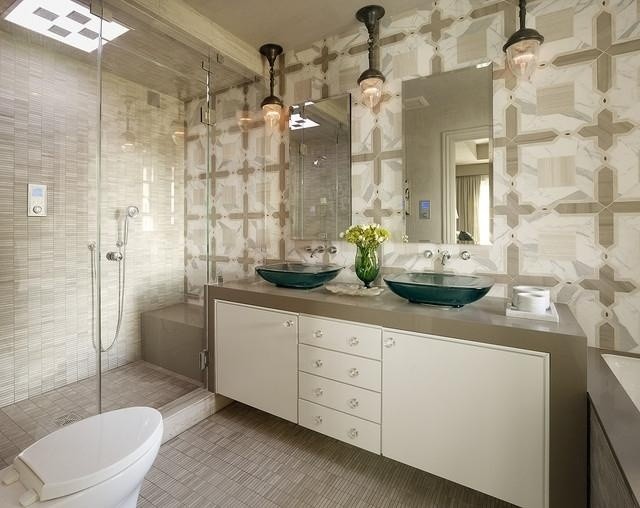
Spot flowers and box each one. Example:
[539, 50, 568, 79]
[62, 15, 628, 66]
[337, 218, 390, 282]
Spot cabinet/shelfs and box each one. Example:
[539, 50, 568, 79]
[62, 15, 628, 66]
[297, 313, 381, 459]
[381, 324, 552, 506]
[211, 298, 299, 427]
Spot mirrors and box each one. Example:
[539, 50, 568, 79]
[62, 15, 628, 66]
[402, 60, 495, 248]
[286, 92, 352, 242]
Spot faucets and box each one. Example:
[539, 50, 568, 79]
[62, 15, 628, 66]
[309, 245, 324, 258]
[437, 248, 449, 265]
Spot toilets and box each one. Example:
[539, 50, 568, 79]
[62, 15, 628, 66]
[0, 406, 170, 507]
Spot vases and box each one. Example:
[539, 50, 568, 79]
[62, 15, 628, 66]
[354, 244, 381, 288]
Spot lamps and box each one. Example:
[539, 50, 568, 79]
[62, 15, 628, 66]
[354, 3, 389, 108]
[258, 41, 286, 129]
[501, 0, 545, 82]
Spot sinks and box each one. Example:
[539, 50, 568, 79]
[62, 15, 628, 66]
[384, 271, 496, 309]
[254, 261, 345, 290]
